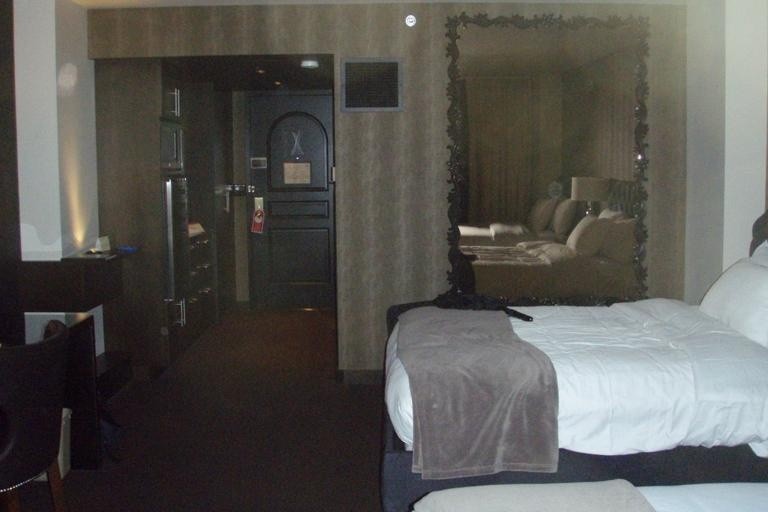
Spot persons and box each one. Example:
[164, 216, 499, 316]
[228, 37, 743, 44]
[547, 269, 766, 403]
[251, 210, 264, 232]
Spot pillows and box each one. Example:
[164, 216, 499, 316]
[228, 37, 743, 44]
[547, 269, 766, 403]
[700, 255, 767, 350]
[529, 197, 638, 264]
[750, 239, 768, 267]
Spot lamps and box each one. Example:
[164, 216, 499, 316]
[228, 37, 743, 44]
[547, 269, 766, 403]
[570, 176, 610, 215]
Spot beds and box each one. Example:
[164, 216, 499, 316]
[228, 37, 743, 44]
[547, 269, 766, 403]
[410, 478, 768, 512]
[459, 239, 641, 299]
[383, 211, 768, 460]
[456, 219, 558, 245]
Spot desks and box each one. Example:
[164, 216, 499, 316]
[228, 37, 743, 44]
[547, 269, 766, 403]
[1, 316, 103, 470]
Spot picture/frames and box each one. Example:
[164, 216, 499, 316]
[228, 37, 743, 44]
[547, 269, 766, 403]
[340, 57, 404, 112]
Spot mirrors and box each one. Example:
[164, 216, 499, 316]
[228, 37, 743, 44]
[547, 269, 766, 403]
[442, 8, 652, 306]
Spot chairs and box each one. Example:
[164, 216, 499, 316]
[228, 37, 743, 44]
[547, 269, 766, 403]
[0, 321, 73, 512]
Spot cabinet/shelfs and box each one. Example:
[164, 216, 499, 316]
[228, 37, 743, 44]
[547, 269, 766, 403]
[93, 57, 219, 364]
